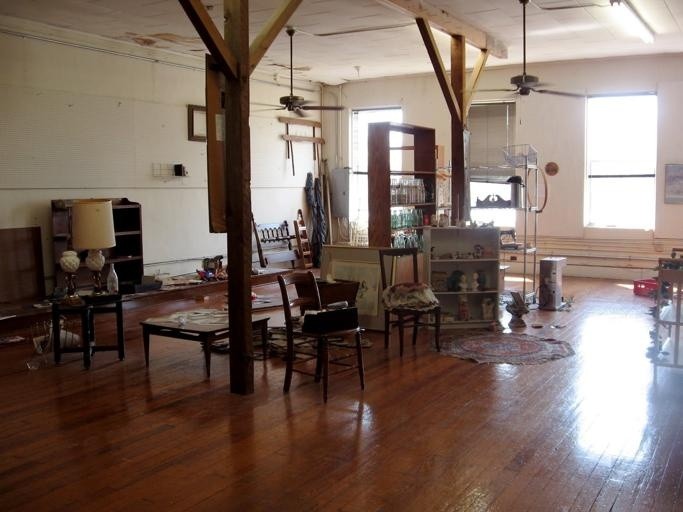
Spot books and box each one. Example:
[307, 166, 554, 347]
[199, 314, 371, 378]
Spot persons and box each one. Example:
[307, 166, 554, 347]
[470, 270, 485, 292]
[448, 271, 469, 290]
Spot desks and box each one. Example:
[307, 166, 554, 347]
[139, 308, 271, 377]
[295, 277, 360, 315]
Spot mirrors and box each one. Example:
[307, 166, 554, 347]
[465, 101, 519, 230]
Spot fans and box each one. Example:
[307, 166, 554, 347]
[249, 29, 345, 118]
[460, 0, 587, 100]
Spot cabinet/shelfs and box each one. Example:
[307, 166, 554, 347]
[423, 225, 500, 330]
[448, 142, 539, 305]
[367, 121, 436, 248]
[50, 197, 144, 297]
[652, 247, 683, 389]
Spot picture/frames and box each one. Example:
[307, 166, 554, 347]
[319, 244, 396, 334]
[187, 104, 207, 142]
[663, 163, 683, 205]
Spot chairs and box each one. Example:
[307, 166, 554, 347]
[277, 270, 366, 404]
[378, 248, 441, 358]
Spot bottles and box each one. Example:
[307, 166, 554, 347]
[390, 177, 426, 249]
[107, 263, 118, 292]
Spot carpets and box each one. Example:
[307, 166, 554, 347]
[426, 330, 575, 365]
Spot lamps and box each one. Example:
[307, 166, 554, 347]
[72, 197, 117, 294]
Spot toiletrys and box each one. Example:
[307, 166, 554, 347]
[389, 177, 435, 252]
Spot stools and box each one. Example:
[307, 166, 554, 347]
[51, 291, 125, 371]
